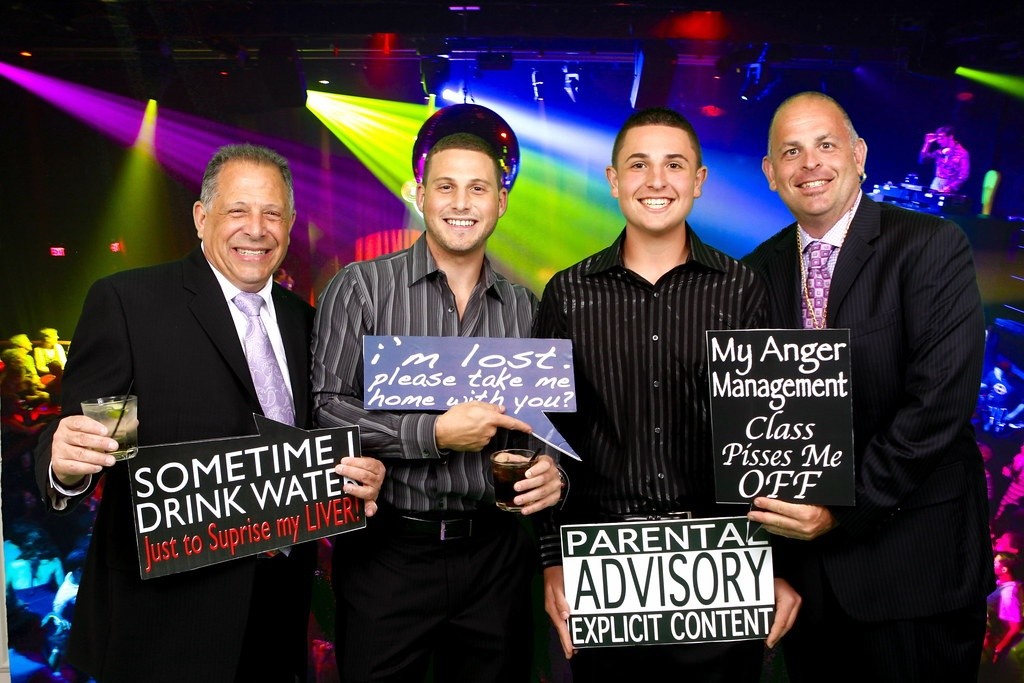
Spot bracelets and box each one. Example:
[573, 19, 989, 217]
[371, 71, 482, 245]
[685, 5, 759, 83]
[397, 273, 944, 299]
[557, 470, 569, 505]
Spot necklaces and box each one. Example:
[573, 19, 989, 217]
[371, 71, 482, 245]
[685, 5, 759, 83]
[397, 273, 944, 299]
[794, 207, 852, 328]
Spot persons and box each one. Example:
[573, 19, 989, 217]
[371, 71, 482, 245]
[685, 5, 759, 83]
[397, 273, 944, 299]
[974, 438, 1024, 683]
[742, 91, 988, 683]
[0, 326, 88, 683]
[310, 101, 571, 682]
[35, 141, 386, 683]
[525, 108, 815, 683]
[921, 123, 971, 197]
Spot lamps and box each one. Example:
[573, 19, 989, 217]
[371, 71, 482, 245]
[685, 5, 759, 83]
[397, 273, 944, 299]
[420, 35, 783, 105]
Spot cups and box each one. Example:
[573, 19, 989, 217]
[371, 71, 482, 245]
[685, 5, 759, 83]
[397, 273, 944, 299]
[489, 449, 538, 512]
[80, 395, 139, 462]
